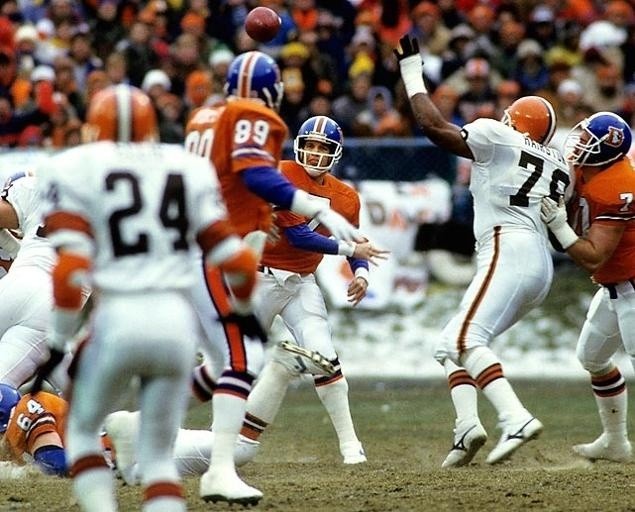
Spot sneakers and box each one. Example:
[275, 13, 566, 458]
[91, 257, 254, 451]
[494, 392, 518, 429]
[442, 414, 488, 470]
[573, 433, 632, 463]
[198, 473, 264, 507]
[339, 440, 368, 464]
[276, 340, 335, 378]
[486, 409, 544, 465]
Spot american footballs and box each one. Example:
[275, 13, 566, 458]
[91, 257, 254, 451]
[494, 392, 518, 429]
[244, 7, 279, 42]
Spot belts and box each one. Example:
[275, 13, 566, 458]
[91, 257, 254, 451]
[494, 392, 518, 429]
[256, 264, 273, 275]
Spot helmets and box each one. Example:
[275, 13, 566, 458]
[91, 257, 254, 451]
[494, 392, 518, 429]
[0, 383, 21, 433]
[80, 83, 158, 142]
[293, 116, 344, 178]
[500, 95, 557, 146]
[222, 51, 285, 112]
[563, 111, 633, 167]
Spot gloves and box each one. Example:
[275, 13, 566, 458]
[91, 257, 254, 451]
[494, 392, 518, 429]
[215, 310, 268, 342]
[319, 210, 369, 246]
[541, 195, 568, 232]
[392, 33, 424, 66]
